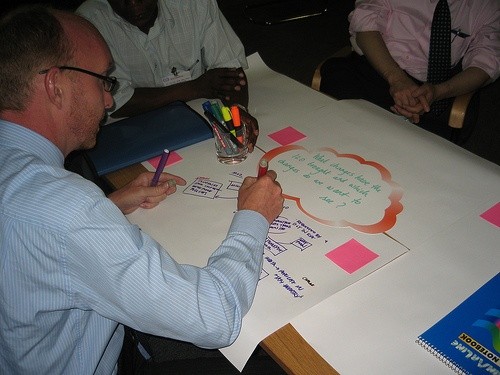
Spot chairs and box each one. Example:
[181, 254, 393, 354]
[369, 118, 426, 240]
[309, 45, 479, 146]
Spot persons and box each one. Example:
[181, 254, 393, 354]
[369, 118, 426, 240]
[347, 0, 496, 123]
[1, 0, 285, 375]
[73, 1, 259, 154]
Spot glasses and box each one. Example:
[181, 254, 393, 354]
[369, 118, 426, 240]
[39, 66, 117, 92]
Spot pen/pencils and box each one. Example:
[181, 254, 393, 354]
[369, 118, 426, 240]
[201, 99, 246, 156]
[255, 160, 267, 181]
[147, 149, 170, 187]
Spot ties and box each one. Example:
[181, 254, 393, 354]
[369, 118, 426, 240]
[426, 0, 452, 113]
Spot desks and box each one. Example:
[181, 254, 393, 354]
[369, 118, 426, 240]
[104, 163, 339, 375]
